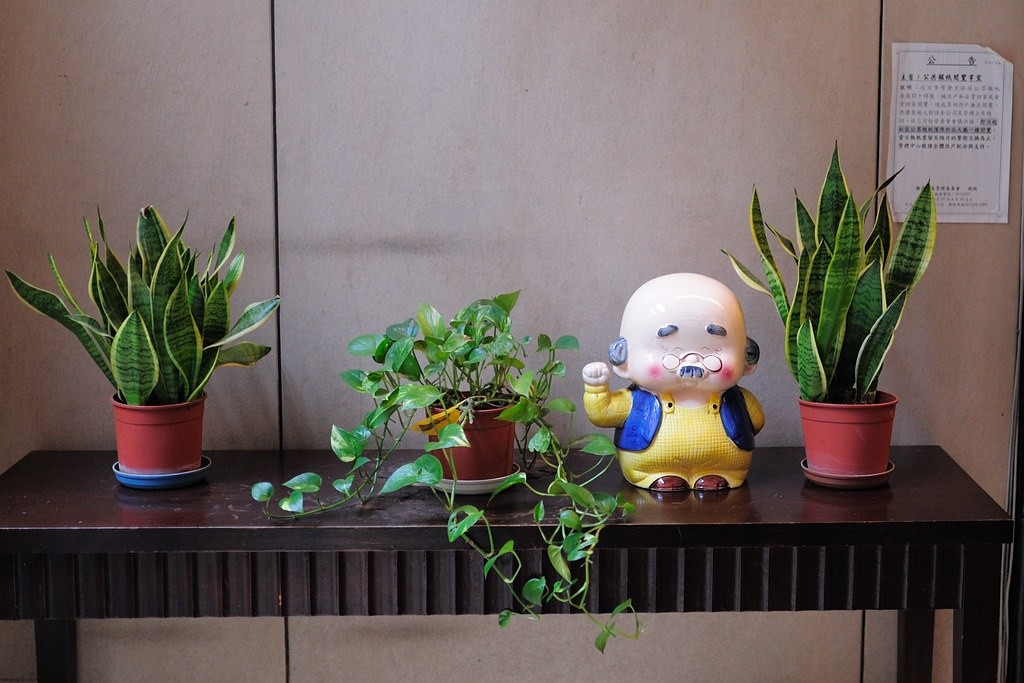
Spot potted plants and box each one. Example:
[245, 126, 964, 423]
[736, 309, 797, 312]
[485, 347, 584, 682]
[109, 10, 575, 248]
[719, 139, 937, 485]
[252, 286, 641, 654]
[3, 205, 281, 489]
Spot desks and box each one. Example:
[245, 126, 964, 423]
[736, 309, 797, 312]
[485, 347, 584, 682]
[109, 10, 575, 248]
[0, 444, 1014, 683]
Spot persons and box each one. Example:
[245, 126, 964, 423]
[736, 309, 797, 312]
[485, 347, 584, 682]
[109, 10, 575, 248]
[583, 274, 766, 491]
